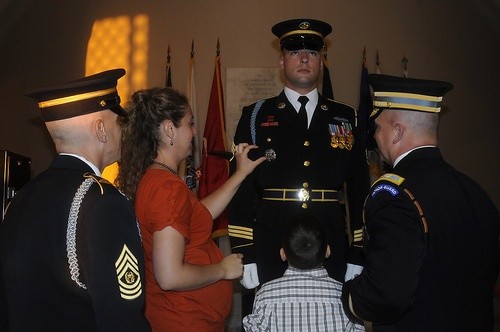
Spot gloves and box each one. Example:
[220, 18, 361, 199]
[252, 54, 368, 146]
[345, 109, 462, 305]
[239, 263, 260, 290]
[345, 263, 364, 282]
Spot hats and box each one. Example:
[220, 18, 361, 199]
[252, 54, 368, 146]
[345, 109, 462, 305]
[367, 74, 454, 120]
[23, 69, 129, 123]
[271, 19, 332, 51]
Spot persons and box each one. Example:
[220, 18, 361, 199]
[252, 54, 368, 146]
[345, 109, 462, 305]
[1, 68, 500, 332]
[235, 18, 372, 319]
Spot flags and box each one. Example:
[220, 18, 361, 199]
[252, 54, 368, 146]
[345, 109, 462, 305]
[359, 63, 379, 153]
[160, 53, 232, 238]
[323, 59, 335, 98]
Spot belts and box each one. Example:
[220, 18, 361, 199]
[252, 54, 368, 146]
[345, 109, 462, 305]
[261, 188, 339, 201]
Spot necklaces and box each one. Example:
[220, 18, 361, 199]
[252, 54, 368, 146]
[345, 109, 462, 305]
[153, 161, 177, 177]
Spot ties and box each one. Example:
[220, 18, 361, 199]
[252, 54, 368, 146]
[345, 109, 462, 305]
[298, 96, 309, 130]
